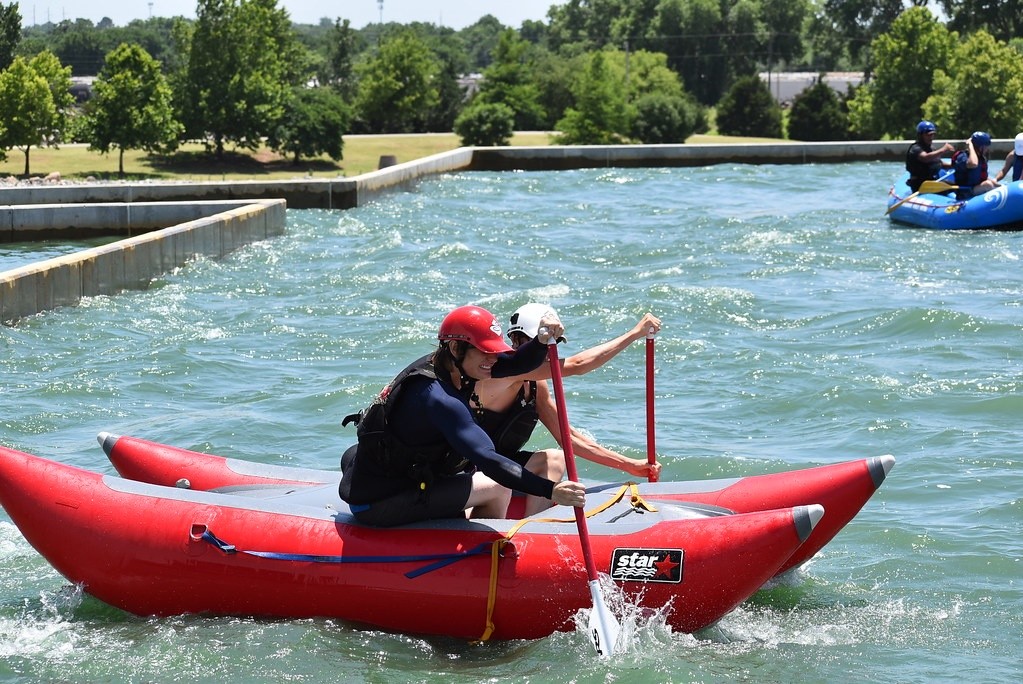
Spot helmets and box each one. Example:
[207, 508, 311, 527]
[506, 302, 567, 344]
[438, 305, 516, 354]
[971, 131, 991, 148]
[1015, 132, 1023, 156]
[916, 121, 935, 132]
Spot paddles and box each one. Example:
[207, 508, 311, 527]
[646, 327, 661, 481]
[885, 171, 955, 216]
[546, 327, 622, 661]
[919, 180, 972, 194]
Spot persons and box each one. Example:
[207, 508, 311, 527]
[906, 122, 956, 196]
[339, 304, 661, 520]
[951, 132, 1002, 200]
[995, 133, 1023, 181]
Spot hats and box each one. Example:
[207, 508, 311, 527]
[920, 130, 936, 135]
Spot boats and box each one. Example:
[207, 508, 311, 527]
[0, 429, 901, 644]
[887, 172, 1023, 231]
